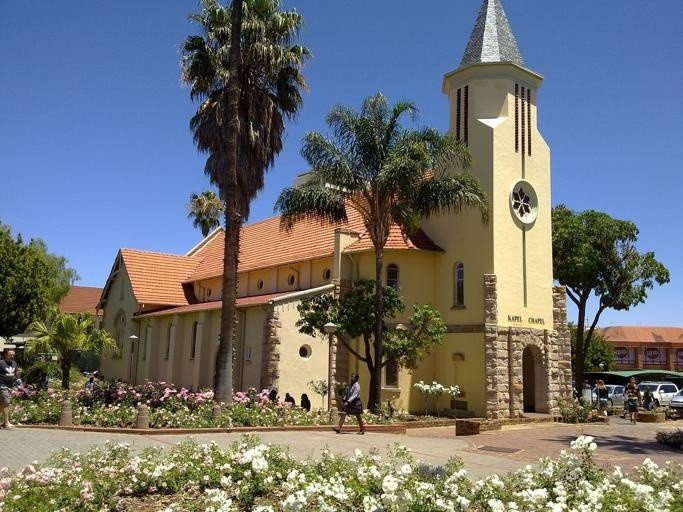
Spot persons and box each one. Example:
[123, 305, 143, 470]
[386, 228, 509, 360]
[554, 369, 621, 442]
[38, 372, 49, 390]
[584, 377, 655, 424]
[81, 365, 127, 389]
[332, 373, 364, 434]
[269, 390, 280, 401]
[301, 394, 310, 411]
[285, 393, 295, 405]
[0, 348, 23, 430]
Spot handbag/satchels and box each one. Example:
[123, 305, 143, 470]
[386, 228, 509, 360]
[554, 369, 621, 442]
[345, 399, 364, 415]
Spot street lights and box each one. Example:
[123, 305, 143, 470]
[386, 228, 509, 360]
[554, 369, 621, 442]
[128, 334, 139, 383]
[324, 320, 339, 417]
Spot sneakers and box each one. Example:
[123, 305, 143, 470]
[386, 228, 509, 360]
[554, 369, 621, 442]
[357, 431, 363, 434]
[332, 427, 340, 433]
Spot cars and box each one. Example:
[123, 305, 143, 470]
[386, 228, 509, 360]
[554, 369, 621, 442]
[591, 384, 627, 407]
[670, 388, 683, 412]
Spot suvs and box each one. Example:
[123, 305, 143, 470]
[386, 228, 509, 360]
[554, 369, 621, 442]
[636, 380, 679, 409]
